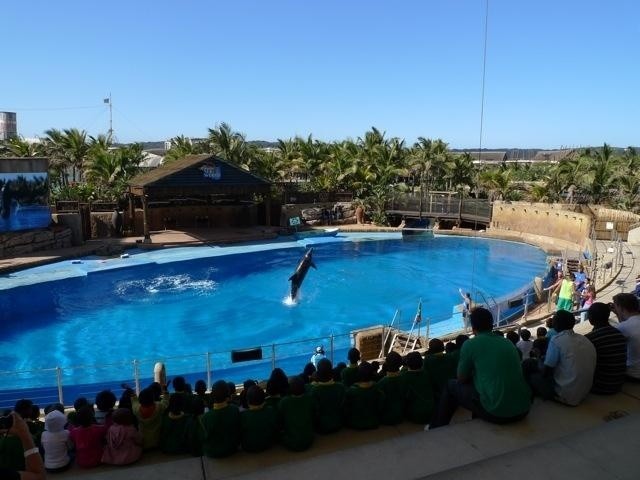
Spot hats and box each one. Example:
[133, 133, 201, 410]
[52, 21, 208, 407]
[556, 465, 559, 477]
[44, 410, 67, 431]
[247, 386, 265, 405]
[77, 408, 94, 426]
[113, 408, 133, 424]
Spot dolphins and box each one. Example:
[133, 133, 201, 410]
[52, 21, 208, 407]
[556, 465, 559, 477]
[288, 247, 317, 300]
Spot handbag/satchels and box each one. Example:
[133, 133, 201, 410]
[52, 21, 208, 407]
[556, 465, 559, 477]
[551, 292, 558, 302]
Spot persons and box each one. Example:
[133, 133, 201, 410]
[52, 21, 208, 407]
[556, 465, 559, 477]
[523, 267, 640, 406]
[458, 288, 470, 329]
[2, 346, 346, 480]
[345, 307, 558, 430]
